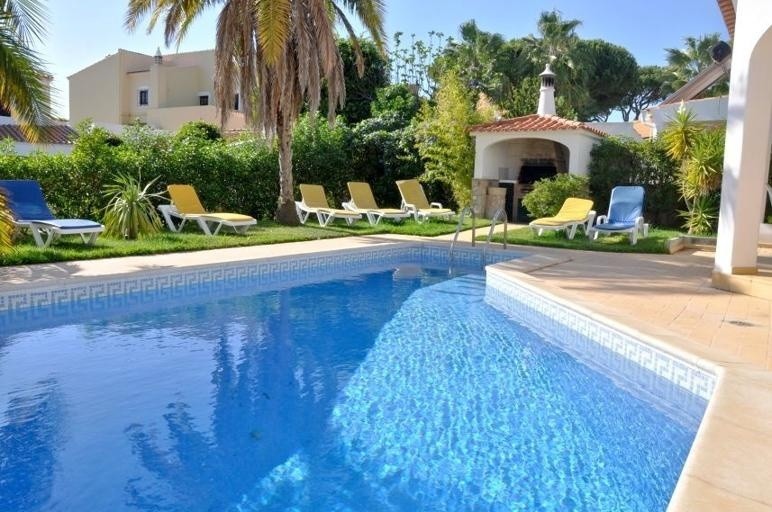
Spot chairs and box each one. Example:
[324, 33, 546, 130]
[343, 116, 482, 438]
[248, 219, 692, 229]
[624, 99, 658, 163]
[529, 185, 650, 246]
[156, 182, 258, 236]
[1, 178, 106, 249]
[293, 178, 456, 227]
